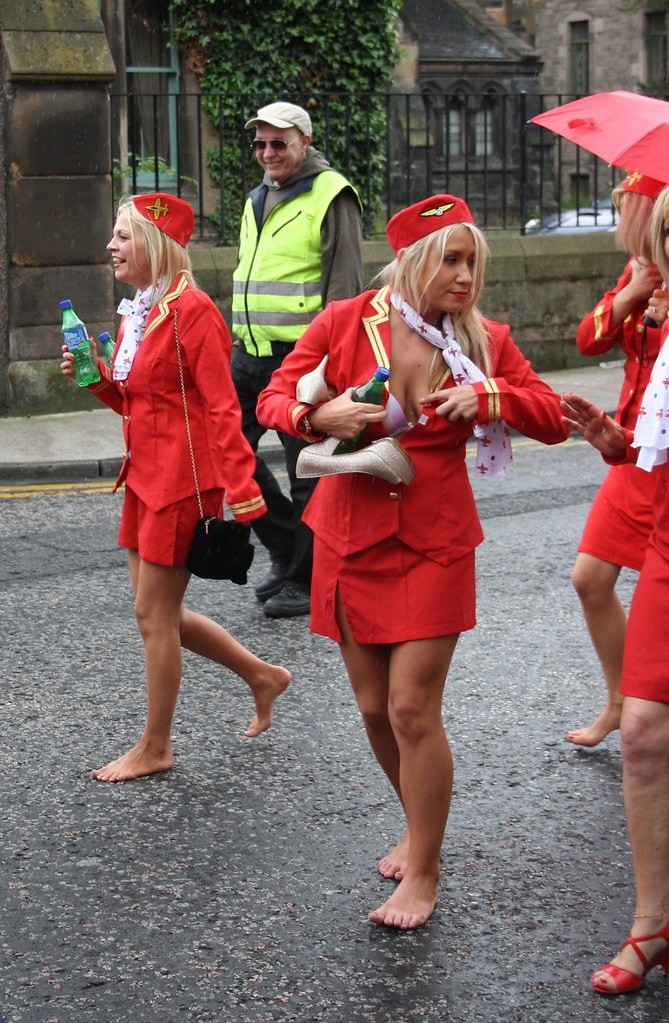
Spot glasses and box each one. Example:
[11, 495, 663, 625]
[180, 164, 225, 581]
[249, 135, 298, 151]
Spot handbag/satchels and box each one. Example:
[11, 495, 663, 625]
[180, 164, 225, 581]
[186, 514, 255, 585]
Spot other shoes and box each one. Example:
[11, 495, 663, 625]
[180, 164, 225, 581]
[263, 582, 310, 618]
[254, 562, 289, 602]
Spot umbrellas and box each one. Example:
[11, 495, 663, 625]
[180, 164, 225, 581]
[527, 90, 669, 186]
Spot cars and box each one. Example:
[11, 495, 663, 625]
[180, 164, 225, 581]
[525, 208, 621, 235]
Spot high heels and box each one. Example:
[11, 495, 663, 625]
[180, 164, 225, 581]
[592, 921, 669, 996]
[296, 435, 416, 487]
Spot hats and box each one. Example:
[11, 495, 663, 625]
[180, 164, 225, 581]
[623, 168, 666, 198]
[133, 193, 194, 248]
[386, 194, 473, 253]
[245, 102, 312, 136]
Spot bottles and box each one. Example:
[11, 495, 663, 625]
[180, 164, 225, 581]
[59, 298, 101, 388]
[332, 366, 390, 455]
[98, 331, 116, 365]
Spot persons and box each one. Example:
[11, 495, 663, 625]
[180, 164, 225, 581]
[254, 193, 567, 932]
[558, 187, 669, 995]
[61, 192, 290, 782]
[231, 102, 362, 615]
[567, 174, 669, 747]
